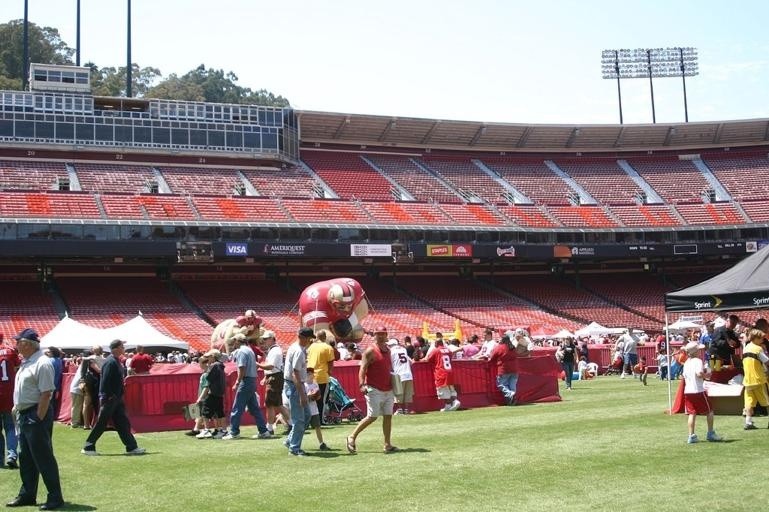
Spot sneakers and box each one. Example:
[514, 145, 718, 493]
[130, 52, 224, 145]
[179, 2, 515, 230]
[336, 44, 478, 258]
[707, 431, 723, 442]
[222, 434, 240, 440]
[743, 424, 757, 429]
[394, 409, 403, 415]
[213, 430, 228, 439]
[441, 404, 451, 411]
[384, 446, 403, 454]
[282, 425, 292, 434]
[688, 433, 702, 444]
[196, 431, 213, 438]
[284, 440, 290, 448]
[404, 409, 409, 414]
[269, 430, 275, 435]
[347, 437, 357, 455]
[289, 448, 307, 456]
[7, 457, 20, 469]
[506, 392, 517, 404]
[71, 423, 82, 427]
[185, 429, 200, 436]
[621, 373, 626, 379]
[450, 399, 460, 411]
[252, 431, 271, 438]
[81, 449, 100, 456]
[212, 429, 220, 435]
[319, 443, 331, 451]
[127, 447, 145, 454]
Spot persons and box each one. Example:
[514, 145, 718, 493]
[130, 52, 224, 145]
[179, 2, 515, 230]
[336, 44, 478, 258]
[70, 355, 100, 427]
[148, 347, 206, 366]
[0, 333, 21, 465]
[80, 340, 147, 455]
[712, 315, 740, 366]
[638, 356, 648, 386]
[345, 326, 402, 454]
[386, 338, 414, 416]
[5, 329, 65, 511]
[594, 329, 618, 344]
[301, 367, 331, 453]
[83, 360, 99, 429]
[526, 327, 562, 350]
[401, 336, 430, 365]
[742, 330, 769, 429]
[755, 319, 769, 355]
[615, 327, 645, 380]
[185, 359, 220, 437]
[557, 338, 579, 392]
[427, 339, 460, 412]
[286, 327, 317, 456]
[330, 339, 361, 363]
[487, 336, 517, 405]
[195, 350, 227, 441]
[302, 330, 334, 433]
[253, 329, 292, 437]
[431, 332, 501, 357]
[43, 347, 62, 421]
[656, 329, 672, 380]
[654, 349, 670, 380]
[514, 330, 528, 355]
[124, 345, 153, 377]
[575, 355, 591, 380]
[702, 321, 717, 363]
[221, 333, 272, 441]
[682, 342, 723, 444]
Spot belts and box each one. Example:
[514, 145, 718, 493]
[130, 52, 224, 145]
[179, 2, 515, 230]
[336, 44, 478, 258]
[15, 405, 38, 415]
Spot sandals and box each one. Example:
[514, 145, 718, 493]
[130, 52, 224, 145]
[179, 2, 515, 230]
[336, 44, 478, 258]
[568, 386, 571, 392]
[84, 425, 93, 430]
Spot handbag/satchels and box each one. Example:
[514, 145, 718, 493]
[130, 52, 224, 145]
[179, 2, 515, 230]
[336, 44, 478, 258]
[558, 344, 570, 364]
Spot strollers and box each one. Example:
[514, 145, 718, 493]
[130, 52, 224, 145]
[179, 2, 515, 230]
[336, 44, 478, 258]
[322, 377, 364, 425]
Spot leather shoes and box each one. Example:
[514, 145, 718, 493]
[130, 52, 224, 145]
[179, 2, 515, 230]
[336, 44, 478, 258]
[39, 500, 63, 510]
[6, 496, 36, 507]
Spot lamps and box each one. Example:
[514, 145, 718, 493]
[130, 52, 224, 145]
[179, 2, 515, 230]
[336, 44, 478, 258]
[601, 47, 701, 80]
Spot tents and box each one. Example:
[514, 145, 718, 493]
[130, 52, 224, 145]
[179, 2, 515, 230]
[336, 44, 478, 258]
[665, 246, 769, 415]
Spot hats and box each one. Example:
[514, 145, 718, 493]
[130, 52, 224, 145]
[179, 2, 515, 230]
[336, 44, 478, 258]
[244, 311, 257, 318]
[298, 328, 318, 340]
[374, 325, 388, 334]
[14, 328, 40, 341]
[204, 350, 222, 359]
[229, 334, 248, 342]
[261, 330, 276, 340]
[110, 340, 127, 350]
[685, 342, 706, 355]
[327, 283, 355, 304]
[385, 339, 399, 346]
[235, 317, 247, 325]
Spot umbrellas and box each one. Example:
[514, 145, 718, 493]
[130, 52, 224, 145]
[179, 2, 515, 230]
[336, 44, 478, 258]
[29, 318, 126, 350]
[101, 317, 189, 352]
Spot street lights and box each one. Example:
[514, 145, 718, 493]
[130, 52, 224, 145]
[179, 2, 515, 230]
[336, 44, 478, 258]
[602, 46, 699, 126]
